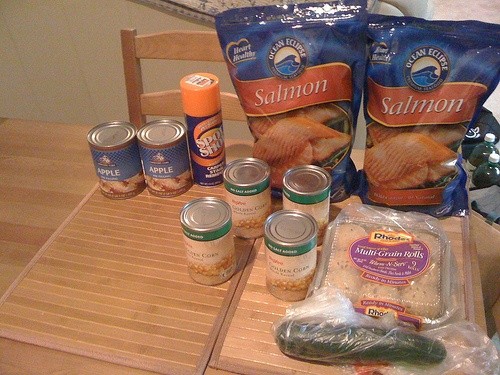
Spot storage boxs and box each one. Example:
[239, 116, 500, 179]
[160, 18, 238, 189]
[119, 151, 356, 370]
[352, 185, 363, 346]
[314, 217, 454, 328]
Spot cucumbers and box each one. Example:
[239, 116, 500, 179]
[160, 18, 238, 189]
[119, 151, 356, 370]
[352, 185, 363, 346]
[275, 319, 447, 365]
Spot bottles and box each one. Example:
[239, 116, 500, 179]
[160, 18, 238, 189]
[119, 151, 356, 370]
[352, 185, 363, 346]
[468, 152, 500, 190]
[180, 73, 227, 188]
[466, 132, 499, 173]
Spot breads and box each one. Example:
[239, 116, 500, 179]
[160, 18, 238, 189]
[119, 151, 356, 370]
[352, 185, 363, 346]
[248, 104, 352, 190]
[363, 123, 464, 189]
[325, 223, 444, 317]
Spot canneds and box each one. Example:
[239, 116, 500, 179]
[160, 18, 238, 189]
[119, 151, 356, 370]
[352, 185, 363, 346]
[137, 119, 194, 198]
[263, 209, 319, 301]
[179, 197, 237, 285]
[87, 120, 146, 199]
[282, 165, 331, 246]
[223, 157, 271, 238]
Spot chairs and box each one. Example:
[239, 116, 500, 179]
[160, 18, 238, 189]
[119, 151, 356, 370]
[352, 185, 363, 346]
[120, 28, 255, 140]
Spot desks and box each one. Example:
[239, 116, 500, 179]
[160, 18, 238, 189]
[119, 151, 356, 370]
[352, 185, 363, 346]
[0, 116, 500, 375]
